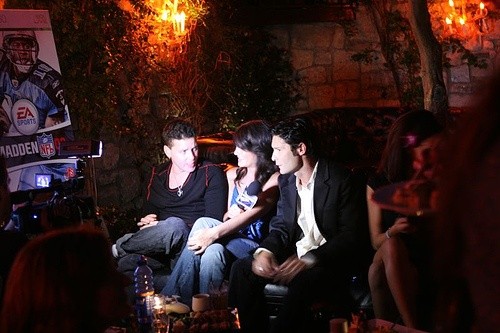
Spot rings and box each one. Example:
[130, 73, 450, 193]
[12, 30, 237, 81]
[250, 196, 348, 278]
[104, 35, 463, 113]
[260, 267, 264, 271]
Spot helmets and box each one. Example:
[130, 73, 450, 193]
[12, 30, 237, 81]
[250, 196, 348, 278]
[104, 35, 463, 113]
[2, 28, 40, 73]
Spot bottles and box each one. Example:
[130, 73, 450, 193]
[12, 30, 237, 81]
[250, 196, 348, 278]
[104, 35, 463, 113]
[133, 255, 156, 323]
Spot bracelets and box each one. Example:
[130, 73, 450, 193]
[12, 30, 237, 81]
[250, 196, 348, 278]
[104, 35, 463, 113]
[385, 228, 392, 240]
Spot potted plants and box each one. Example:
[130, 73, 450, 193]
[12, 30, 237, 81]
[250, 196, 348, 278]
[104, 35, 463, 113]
[196, 30, 306, 154]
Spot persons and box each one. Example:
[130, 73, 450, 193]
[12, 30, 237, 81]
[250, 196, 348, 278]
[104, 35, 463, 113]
[0, 71, 500, 333]
[0, 31, 76, 190]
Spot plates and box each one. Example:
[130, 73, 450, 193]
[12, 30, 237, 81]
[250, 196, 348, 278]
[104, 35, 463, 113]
[370, 179, 436, 217]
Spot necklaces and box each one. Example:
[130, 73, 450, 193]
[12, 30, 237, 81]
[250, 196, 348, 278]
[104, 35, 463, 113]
[172, 169, 188, 197]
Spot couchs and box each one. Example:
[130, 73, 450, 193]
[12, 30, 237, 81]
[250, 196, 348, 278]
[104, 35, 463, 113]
[147, 107, 411, 322]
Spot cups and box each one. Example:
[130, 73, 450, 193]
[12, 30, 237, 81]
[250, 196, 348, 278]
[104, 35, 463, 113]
[145, 294, 166, 319]
[152, 314, 169, 333]
[208, 280, 230, 309]
[192, 294, 210, 312]
